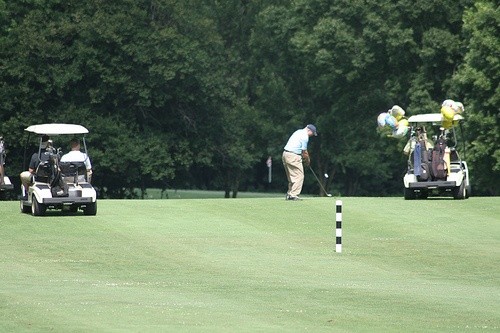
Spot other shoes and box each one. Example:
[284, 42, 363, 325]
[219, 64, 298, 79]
[286, 195, 302, 200]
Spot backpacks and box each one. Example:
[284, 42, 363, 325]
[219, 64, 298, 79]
[430, 138, 451, 180]
[409, 139, 429, 181]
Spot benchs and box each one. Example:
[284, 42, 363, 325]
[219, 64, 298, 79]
[35, 161, 88, 184]
[411, 149, 462, 169]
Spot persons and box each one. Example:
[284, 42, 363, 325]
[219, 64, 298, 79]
[20, 136, 57, 201]
[281, 124, 317, 200]
[60, 137, 91, 183]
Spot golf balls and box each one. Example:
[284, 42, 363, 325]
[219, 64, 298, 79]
[324, 173, 328, 178]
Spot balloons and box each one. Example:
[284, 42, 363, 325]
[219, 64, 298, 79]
[377, 106, 410, 138]
[441, 100, 465, 129]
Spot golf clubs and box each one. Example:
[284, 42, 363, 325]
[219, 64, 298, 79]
[44, 145, 63, 162]
[308, 165, 332, 197]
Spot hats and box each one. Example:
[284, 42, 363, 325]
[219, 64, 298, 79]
[378, 105, 410, 143]
[440, 99, 465, 130]
[20, 192, 28, 200]
[307, 124, 317, 137]
[40, 136, 50, 149]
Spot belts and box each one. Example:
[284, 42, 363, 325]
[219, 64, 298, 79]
[283, 149, 301, 155]
